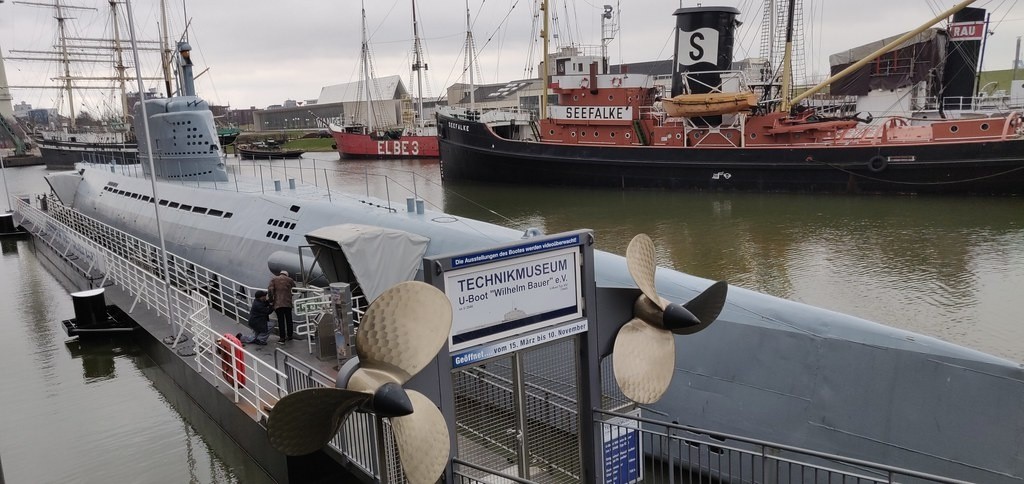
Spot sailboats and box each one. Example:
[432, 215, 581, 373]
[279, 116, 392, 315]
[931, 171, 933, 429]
[306, 0, 619, 162]
[12, 0, 240, 175]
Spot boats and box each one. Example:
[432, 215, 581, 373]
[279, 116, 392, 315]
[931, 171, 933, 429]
[657, 91, 758, 118]
[237, 137, 306, 160]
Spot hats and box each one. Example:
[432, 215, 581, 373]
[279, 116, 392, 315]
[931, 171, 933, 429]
[281, 270, 288, 277]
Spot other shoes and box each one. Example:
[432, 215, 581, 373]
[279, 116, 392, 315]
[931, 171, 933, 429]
[288, 337, 292, 341]
[236, 333, 242, 340]
[254, 338, 267, 345]
[277, 338, 285, 344]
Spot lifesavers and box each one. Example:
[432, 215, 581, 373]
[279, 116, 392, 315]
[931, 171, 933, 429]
[612, 76, 622, 87]
[867, 156, 888, 175]
[579, 78, 590, 88]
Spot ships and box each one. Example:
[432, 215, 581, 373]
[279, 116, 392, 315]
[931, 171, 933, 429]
[42, 40, 1024, 483]
[432, 2, 1024, 195]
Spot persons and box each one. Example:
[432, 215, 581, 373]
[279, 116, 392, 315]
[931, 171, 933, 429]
[927, 68, 946, 119]
[346, 127, 360, 135]
[235, 271, 297, 345]
[371, 130, 400, 141]
[42, 193, 55, 210]
